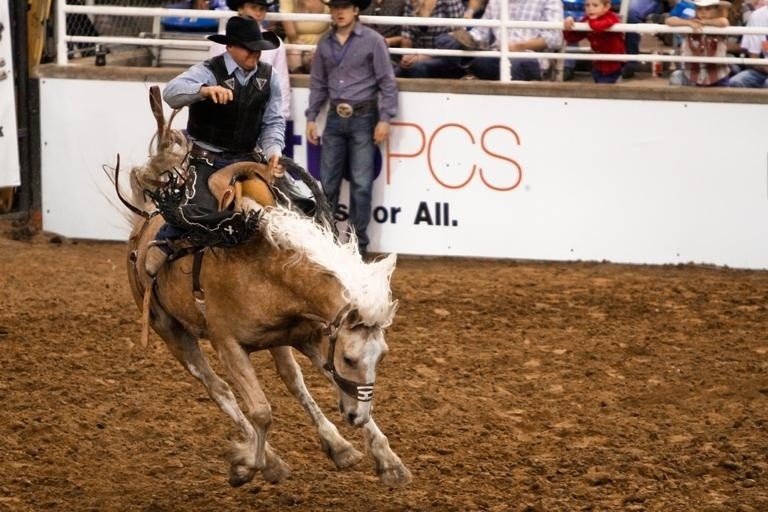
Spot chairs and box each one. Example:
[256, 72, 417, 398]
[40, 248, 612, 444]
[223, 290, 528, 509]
[138, 0, 226, 67]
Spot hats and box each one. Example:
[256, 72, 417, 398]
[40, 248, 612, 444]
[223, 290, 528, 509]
[321, 0, 371, 11]
[683, 0, 731, 8]
[207, 15, 279, 50]
[226, 0, 279, 11]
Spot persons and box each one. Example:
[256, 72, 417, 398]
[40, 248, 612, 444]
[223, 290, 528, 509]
[178, 0, 768, 93]
[207, 0, 291, 122]
[304, 0, 400, 256]
[144, 14, 288, 277]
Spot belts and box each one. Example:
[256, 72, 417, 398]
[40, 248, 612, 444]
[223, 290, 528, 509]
[190, 145, 255, 161]
[330, 100, 373, 118]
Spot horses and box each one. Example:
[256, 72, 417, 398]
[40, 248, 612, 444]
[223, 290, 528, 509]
[94, 87, 414, 489]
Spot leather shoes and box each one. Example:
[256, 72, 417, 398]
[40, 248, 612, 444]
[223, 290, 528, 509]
[144, 246, 169, 278]
